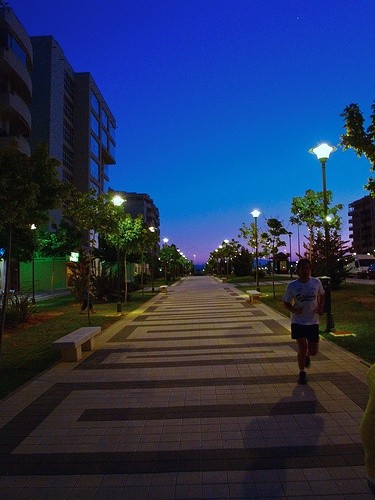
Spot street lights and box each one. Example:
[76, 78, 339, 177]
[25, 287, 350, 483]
[148, 225, 158, 293]
[251, 208, 262, 294]
[218, 245, 223, 279]
[310, 140, 336, 335]
[163, 238, 169, 287]
[207, 249, 218, 276]
[111, 194, 127, 315]
[288, 231, 293, 275]
[223, 238, 231, 278]
[176, 248, 187, 280]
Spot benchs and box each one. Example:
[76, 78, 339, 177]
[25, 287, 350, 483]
[52, 327, 102, 362]
[221, 277, 226, 283]
[160, 285, 168, 293]
[246, 290, 262, 304]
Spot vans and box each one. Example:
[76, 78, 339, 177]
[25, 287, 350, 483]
[343, 253, 375, 278]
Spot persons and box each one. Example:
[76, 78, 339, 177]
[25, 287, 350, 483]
[283, 259, 326, 385]
[79, 287, 98, 314]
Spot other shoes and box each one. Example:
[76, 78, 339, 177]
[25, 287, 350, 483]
[298, 372, 307, 384]
[304, 356, 311, 367]
